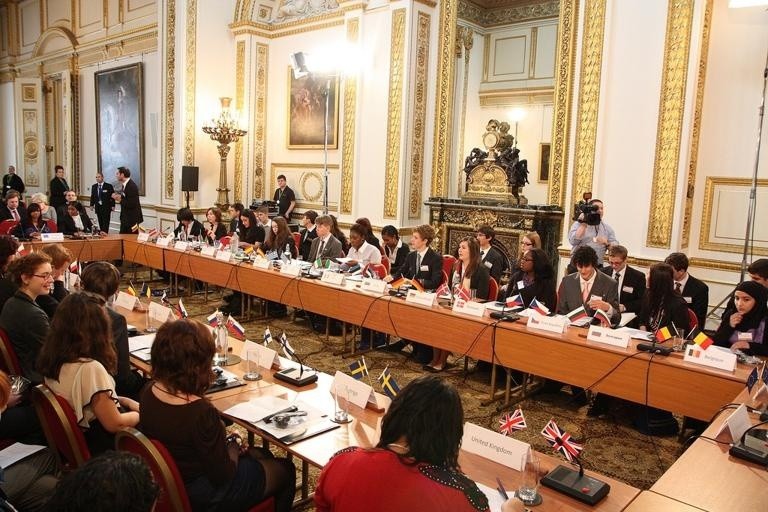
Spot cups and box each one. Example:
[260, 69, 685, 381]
[519, 454, 542, 505]
[468, 288, 477, 304]
[246, 348, 262, 379]
[63, 269, 115, 310]
[144, 305, 159, 334]
[673, 325, 685, 352]
[332, 386, 352, 421]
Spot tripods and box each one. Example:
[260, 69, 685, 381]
[706, 51, 768, 319]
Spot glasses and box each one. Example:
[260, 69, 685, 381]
[608, 259, 625, 265]
[24, 271, 54, 281]
[520, 240, 535, 247]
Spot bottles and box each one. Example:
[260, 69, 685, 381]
[91, 218, 100, 238]
[178, 225, 463, 302]
[212, 312, 230, 366]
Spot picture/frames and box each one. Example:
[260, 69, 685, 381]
[536, 142, 549, 184]
[94, 62, 146, 196]
[285, 62, 339, 149]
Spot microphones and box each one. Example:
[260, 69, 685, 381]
[301, 252, 329, 280]
[637, 308, 674, 356]
[539, 437, 611, 506]
[389, 267, 412, 297]
[243, 242, 263, 260]
[152, 227, 170, 243]
[19, 222, 32, 242]
[194, 225, 202, 252]
[729, 419, 768, 467]
[272, 336, 318, 388]
[162, 298, 187, 319]
[490, 285, 520, 322]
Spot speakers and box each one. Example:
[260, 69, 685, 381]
[287, 223, 299, 233]
[182, 166, 199, 191]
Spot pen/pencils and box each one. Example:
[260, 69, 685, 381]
[496, 477, 509, 500]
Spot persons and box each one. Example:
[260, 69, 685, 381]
[113, 91, 135, 166]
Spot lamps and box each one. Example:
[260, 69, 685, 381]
[202, 96, 247, 211]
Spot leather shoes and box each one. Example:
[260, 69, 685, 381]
[422, 361, 449, 373]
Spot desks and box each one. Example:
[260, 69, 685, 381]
[15, 230, 768, 512]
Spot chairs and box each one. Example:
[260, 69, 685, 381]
[40, 217, 57, 233]
[0, 218, 19, 236]
[0, 329, 24, 378]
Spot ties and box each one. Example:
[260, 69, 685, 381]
[307, 230, 310, 237]
[417, 253, 421, 273]
[480, 250, 484, 258]
[184, 224, 189, 233]
[99, 184, 102, 196]
[614, 272, 621, 285]
[581, 282, 591, 305]
[122, 184, 124, 191]
[317, 240, 326, 257]
[675, 282, 682, 293]
[12, 210, 20, 222]
